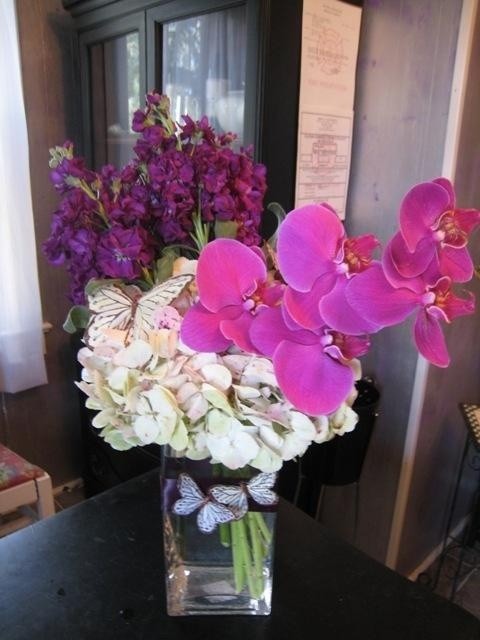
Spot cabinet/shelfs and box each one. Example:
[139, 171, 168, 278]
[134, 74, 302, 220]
[50, 3, 301, 258]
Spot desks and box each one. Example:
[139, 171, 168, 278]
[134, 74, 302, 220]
[1, 452, 476, 636]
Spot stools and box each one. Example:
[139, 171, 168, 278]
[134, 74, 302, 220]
[0, 441, 58, 535]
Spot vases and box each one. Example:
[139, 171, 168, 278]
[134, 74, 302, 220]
[160, 445, 277, 618]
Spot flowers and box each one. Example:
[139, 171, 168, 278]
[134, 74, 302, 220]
[32, 83, 479, 593]
